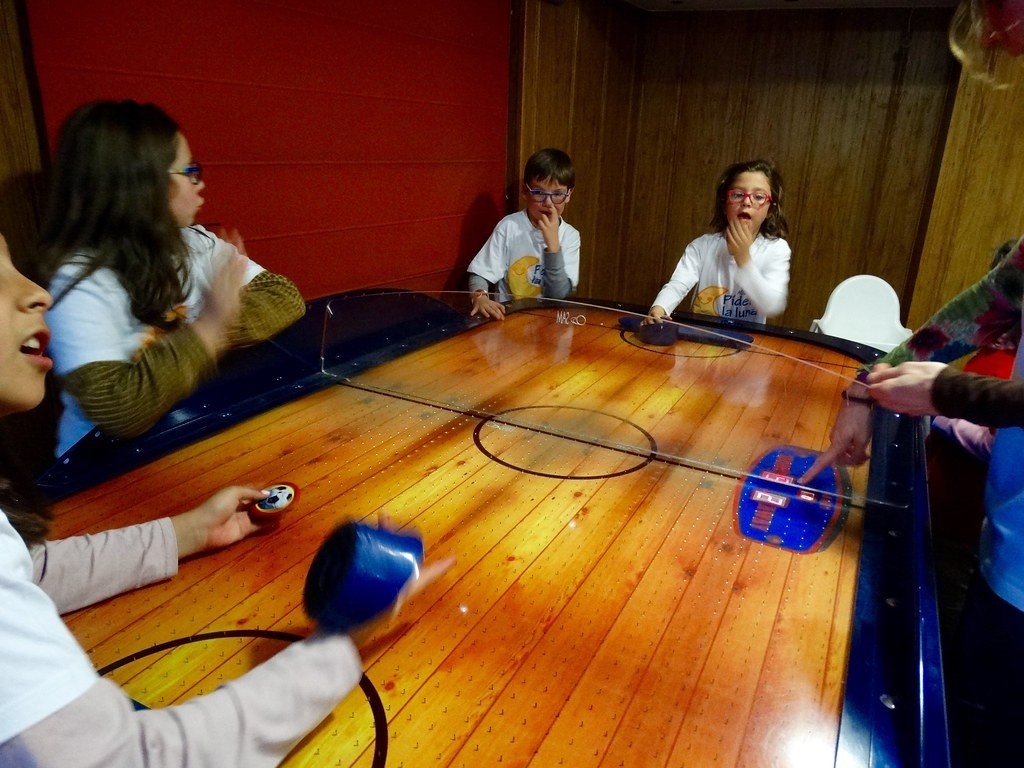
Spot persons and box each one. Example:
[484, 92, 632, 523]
[795, 0, 1024, 768]
[636, 158, 791, 328]
[464, 146, 581, 319]
[43, 100, 311, 491]
[0, 224, 457, 768]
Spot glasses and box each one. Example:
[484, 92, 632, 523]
[525, 182, 571, 204]
[167, 161, 204, 183]
[726, 189, 774, 206]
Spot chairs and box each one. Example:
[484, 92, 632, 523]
[808, 275, 915, 355]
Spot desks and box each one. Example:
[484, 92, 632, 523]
[1, 294, 950, 768]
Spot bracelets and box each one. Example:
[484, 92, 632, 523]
[841, 390, 877, 404]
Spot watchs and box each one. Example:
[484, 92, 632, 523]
[470, 289, 490, 304]
